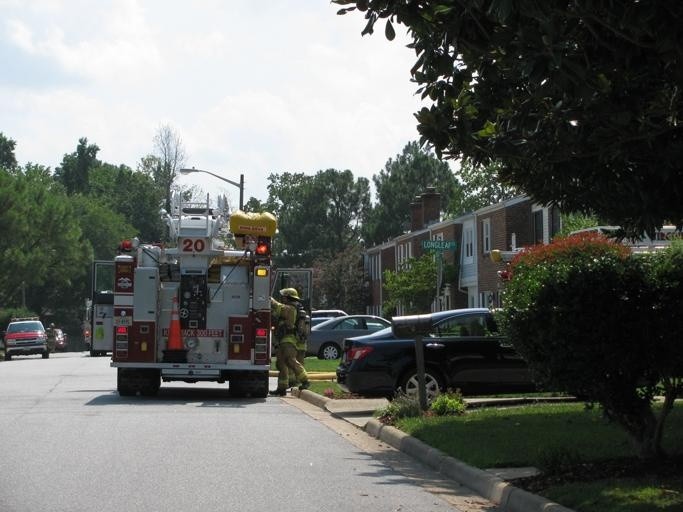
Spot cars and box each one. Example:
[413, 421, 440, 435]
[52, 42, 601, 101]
[44, 323, 68, 353]
[333, 308, 584, 403]
[269, 309, 433, 362]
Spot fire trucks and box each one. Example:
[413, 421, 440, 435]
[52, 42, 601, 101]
[87, 191, 319, 399]
[490, 224, 682, 282]
[78, 289, 114, 356]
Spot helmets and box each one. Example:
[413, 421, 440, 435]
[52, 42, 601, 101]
[280, 287, 300, 300]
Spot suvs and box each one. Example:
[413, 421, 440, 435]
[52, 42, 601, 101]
[0, 317, 52, 360]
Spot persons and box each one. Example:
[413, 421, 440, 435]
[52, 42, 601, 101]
[269, 285, 311, 398]
[43, 321, 57, 352]
[288, 304, 311, 389]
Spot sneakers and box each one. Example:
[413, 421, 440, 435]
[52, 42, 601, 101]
[298, 379, 309, 390]
[269, 387, 286, 396]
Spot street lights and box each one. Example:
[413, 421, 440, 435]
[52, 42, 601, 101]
[177, 165, 246, 220]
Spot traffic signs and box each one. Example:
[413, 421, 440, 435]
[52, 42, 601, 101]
[420, 239, 458, 251]
